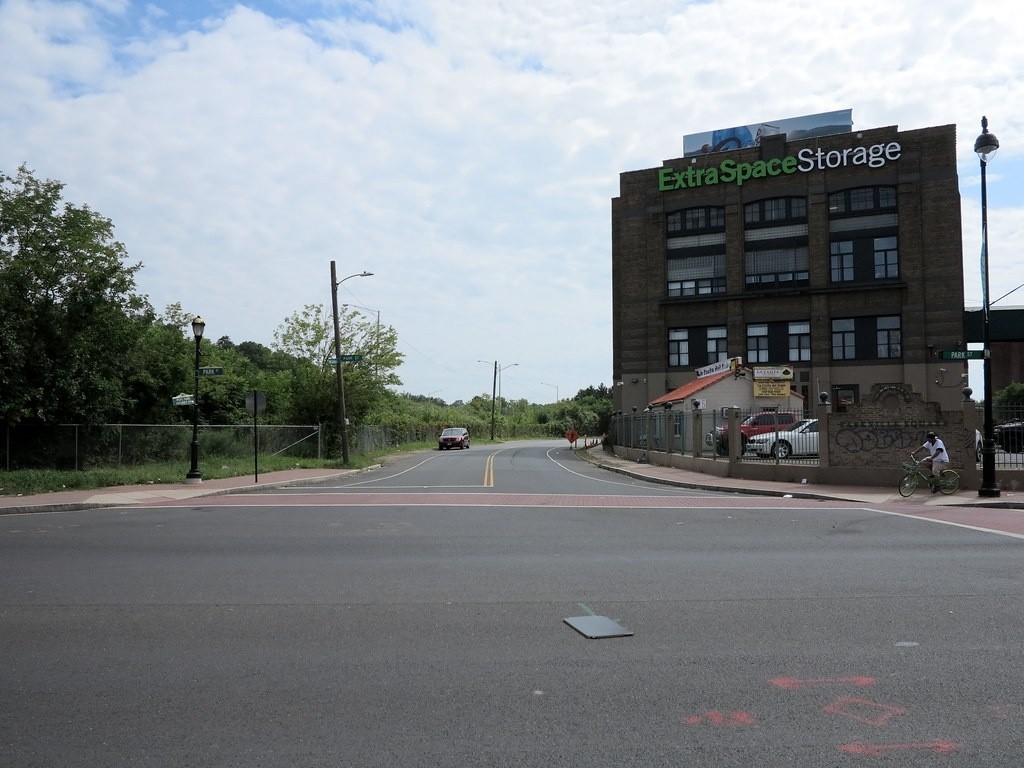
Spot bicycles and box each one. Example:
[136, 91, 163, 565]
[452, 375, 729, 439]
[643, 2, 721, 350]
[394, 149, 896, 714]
[898, 452, 962, 499]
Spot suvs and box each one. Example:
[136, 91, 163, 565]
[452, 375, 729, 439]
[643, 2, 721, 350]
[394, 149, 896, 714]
[705, 411, 799, 456]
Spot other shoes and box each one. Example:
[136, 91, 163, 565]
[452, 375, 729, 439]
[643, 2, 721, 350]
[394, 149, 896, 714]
[931, 486, 942, 494]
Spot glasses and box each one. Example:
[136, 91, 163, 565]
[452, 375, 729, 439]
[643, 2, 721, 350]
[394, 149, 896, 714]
[926, 434, 935, 438]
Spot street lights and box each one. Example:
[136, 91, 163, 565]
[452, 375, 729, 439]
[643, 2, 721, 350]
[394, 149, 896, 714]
[183, 315, 206, 485]
[541, 382, 558, 403]
[477, 359, 501, 412]
[489, 363, 519, 440]
[330, 271, 376, 463]
[975, 113, 1001, 497]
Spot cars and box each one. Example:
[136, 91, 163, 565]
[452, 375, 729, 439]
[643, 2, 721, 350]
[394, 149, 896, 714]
[975, 428, 985, 462]
[994, 421, 1024, 453]
[746, 418, 820, 460]
[438, 427, 471, 450]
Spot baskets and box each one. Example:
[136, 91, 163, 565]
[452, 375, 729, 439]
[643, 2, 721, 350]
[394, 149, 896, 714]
[900, 461, 920, 476]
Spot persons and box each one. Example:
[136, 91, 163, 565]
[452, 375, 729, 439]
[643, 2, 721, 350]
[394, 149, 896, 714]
[910, 432, 949, 494]
